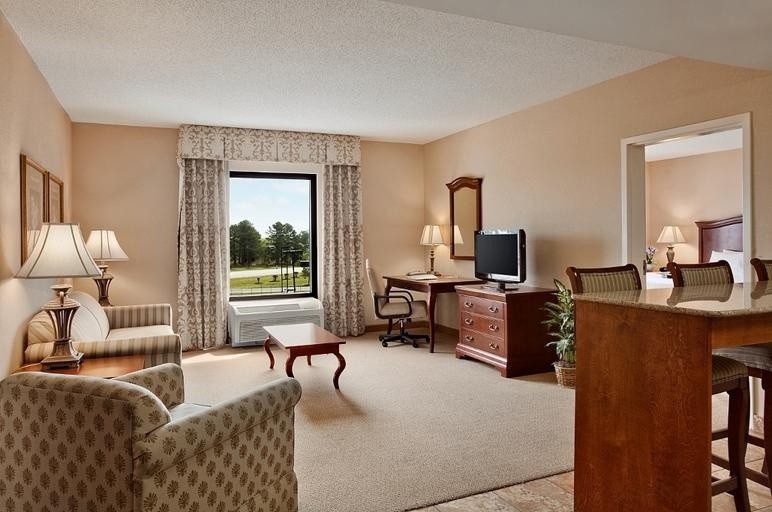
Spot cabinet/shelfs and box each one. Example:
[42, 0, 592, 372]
[454, 284, 561, 378]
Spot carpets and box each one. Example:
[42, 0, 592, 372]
[181, 327, 730, 512]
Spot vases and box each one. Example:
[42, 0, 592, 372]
[646, 263, 654, 272]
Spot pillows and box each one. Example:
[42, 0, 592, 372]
[723, 249, 743, 255]
[709, 250, 741, 268]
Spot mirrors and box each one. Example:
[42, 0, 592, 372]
[446, 176, 484, 261]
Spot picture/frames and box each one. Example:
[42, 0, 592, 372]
[20, 153, 49, 265]
[48, 171, 65, 224]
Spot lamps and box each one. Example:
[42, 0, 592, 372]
[85, 229, 130, 307]
[11, 222, 104, 374]
[453, 224, 464, 244]
[419, 224, 445, 277]
[656, 225, 686, 272]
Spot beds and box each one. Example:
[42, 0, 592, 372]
[646, 271, 744, 290]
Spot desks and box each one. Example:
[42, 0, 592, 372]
[571, 280, 772, 512]
[382, 270, 487, 354]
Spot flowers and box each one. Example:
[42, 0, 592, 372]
[646, 240, 656, 264]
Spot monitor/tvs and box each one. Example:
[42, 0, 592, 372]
[474, 229, 526, 293]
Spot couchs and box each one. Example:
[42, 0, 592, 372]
[24, 289, 182, 369]
[0, 362, 303, 512]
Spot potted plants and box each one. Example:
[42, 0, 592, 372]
[539, 278, 576, 390]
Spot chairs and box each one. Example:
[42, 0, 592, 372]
[667, 259, 772, 490]
[365, 258, 430, 349]
[566, 264, 752, 512]
[750, 257, 772, 282]
